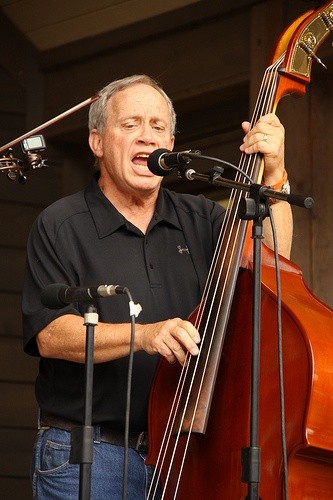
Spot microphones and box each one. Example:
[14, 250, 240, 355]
[40, 283, 128, 309]
[147, 148, 201, 176]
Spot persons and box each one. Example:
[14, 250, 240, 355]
[22, 74, 294, 500]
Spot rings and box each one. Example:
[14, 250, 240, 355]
[263, 134, 267, 141]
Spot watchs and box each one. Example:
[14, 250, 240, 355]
[270, 180, 291, 206]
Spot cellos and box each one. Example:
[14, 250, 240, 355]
[144, 0, 333, 500]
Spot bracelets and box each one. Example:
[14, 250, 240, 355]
[261, 169, 287, 191]
